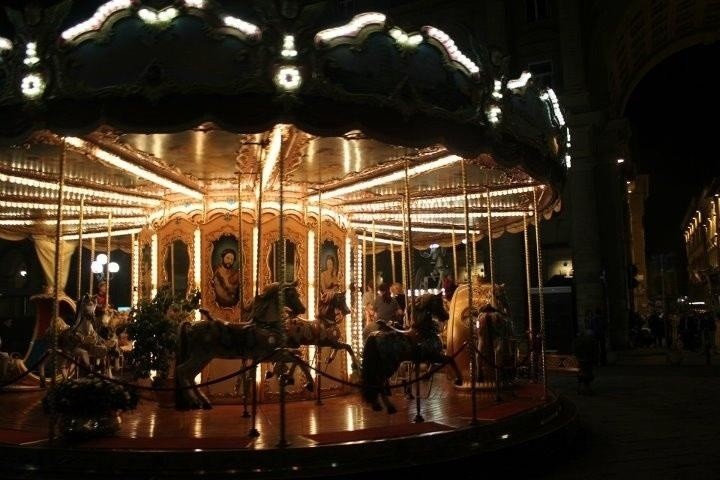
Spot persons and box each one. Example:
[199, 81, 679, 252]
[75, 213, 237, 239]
[213, 248, 241, 307]
[630, 311, 717, 353]
[442, 271, 458, 301]
[320, 255, 340, 291]
[98, 281, 116, 309]
[362, 280, 407, 339]
[582, 308, 609, 368]
